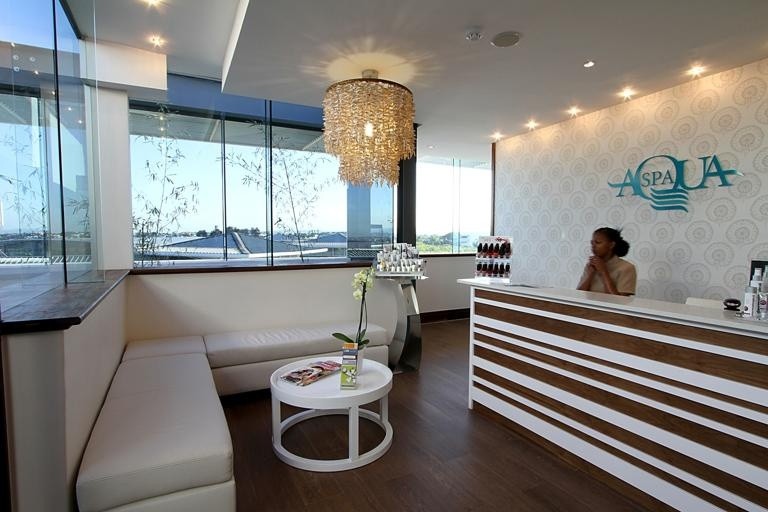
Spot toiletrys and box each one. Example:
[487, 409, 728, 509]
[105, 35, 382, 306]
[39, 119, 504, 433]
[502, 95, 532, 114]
[743, 264, 768, 321]
[376, 243, 425, 273]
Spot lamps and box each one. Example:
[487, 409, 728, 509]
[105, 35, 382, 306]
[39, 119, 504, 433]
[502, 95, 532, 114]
[321, 69, 417, 188]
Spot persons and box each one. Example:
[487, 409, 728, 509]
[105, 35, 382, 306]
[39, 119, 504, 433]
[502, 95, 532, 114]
[574, 226, 638, 297]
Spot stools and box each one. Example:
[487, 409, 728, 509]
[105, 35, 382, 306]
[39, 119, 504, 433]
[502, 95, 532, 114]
[119, 333, 206, 359]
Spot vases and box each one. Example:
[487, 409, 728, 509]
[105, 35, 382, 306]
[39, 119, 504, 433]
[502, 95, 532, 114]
[342, 346, 366, 376]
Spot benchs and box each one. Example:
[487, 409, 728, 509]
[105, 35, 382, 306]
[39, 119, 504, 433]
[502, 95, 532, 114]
[76, 353, 238, 512]
[203, 321, 390, 398]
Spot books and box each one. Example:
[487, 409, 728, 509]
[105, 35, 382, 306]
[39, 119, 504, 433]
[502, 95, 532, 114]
[342, 354, 356, 360]
[341, 346, 358, 354]
[343, 342, 358, 349]
[340, 364, 356, 387]
[342, 359, 357, 365]
[280, 362, 332, 389]
[314, 359, 341, 372]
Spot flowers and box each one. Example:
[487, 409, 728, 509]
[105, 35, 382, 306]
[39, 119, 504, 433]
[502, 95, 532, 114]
[331, 267, 375, 345]
